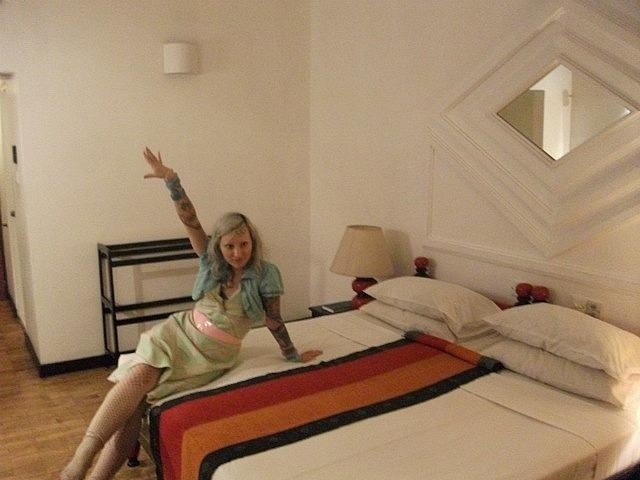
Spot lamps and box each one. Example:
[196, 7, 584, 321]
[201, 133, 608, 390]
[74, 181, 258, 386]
[329, 225, 395, 310]
[163, 42, 197, 74]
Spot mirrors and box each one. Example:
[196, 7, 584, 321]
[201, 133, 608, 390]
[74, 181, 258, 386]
[420, 0, 640, 259]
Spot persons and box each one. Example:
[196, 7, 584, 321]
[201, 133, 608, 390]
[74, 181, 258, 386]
[57, 147, 324, 480]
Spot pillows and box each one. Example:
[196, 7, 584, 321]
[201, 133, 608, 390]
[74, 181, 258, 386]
[357, 276, 640, 410]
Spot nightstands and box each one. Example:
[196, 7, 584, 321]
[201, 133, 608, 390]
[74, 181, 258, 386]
[309, 301, 352, 318]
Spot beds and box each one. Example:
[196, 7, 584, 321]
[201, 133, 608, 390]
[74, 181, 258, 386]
[108, 256, 640, 480]
